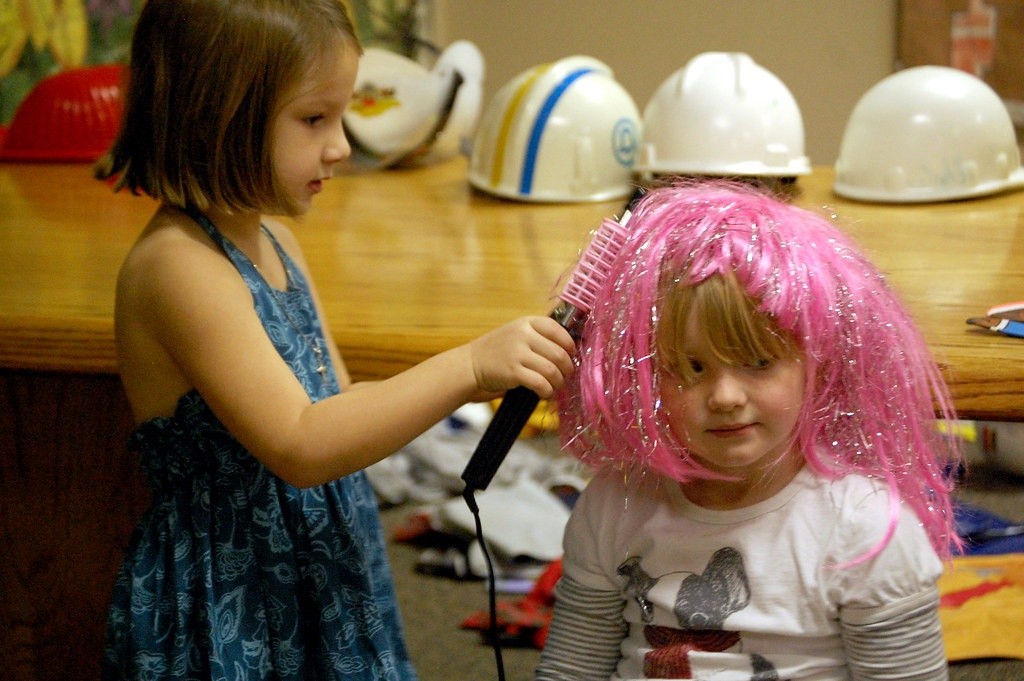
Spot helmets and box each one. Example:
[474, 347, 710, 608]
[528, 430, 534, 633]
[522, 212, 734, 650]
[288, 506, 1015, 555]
[467, 54, 640, 203]
[0, 65, 135, 158]
[831, 64, 1024, 203]
[341, 35, 485, 168]
[633, 51, 812, 176]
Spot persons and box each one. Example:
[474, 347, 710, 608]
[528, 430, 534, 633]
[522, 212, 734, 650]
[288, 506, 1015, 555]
[95, 1, 587, 681]
[522, 178, 950, 681]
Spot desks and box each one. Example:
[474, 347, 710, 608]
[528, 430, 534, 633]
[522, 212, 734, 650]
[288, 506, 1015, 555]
[0, 156, 1024, 422]
